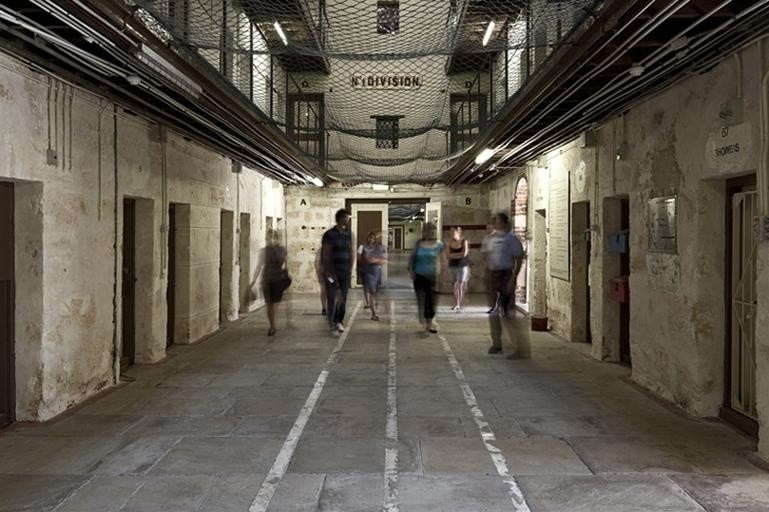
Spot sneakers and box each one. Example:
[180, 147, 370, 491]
[509, 350, 530, 360]
[488, 345, 502, 353]
[321, 308, 327, 314]
[268, 327, 277, 336]
[372, 312, 379, 320]
[331, 322, 345, 338]
[426, 326, 439, 333]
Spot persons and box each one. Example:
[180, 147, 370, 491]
[245, 229, 288, 336]
[407, 211, 532, 360]
[314, 208, 393, 339]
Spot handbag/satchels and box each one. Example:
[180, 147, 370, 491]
[273, 270, 291, 288]
